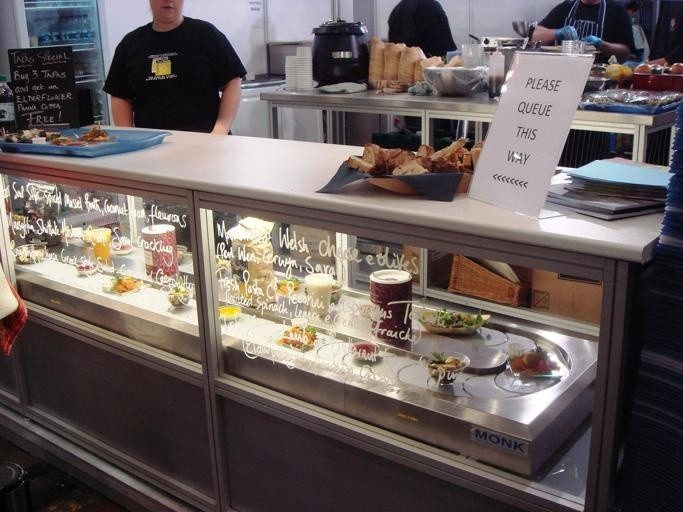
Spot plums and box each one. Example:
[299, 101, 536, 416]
[650, 67, 672, 74]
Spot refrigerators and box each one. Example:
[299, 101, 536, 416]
[0, 0, 114, 127]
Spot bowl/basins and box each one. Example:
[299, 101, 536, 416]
[506, 342, 526, 355]
[112, 245, 133, 255]
[419, 350, 471, 386]
[76, 263, 98, 275]
[423, 67, 488, 97]
[419, 310, 491, 336]
[584, 77, 611, 92]
[512, 20, 538, 38]
[14, 243, 46, 264]
[218, 305, 242, 322]
[160, 283, 196, 308]
[331, 283, 343, 306]
[348, 340, 380, 361]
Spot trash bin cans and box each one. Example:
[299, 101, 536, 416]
[0, 462, 32, 512]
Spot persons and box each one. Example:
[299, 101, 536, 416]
[528, 0, 683, 66]
[102, 0, 245, 134]
[0, 174, 71, 512]
[389, 0, 457, 62]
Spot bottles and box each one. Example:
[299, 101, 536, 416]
[488, 45, 505, 98]
[0, 74, 15, 133]
[33, 14, 92, 48]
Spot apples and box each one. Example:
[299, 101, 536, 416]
[638, 64, 650, 73]
[672, 62, 683, 73]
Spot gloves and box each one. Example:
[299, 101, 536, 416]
[555, 26, 601, 48]
[623, 61, 645, 69]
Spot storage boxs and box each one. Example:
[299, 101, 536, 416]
[531, 269, 604, 324]
[448, 255, 531, 307]
[403, 244, 453, 286]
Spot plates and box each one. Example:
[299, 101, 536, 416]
[501, 348, 564, 379]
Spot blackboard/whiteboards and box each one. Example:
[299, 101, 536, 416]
[8, 46, 80, 130]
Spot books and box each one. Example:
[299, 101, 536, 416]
[544, 157, 672, 220]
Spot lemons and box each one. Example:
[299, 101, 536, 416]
[606, 63, 631, 79]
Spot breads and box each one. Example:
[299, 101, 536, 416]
[349, 135, 484, 176]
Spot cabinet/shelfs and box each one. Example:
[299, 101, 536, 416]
[0, 128, 664, 512]
[258, 83, 677, 290]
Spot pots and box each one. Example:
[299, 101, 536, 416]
[312, 16, 369, 88]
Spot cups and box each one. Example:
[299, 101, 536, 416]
[91, 227, 111, 261]
[285, 47, 313, 91]
[305, 273, 333, 318]
